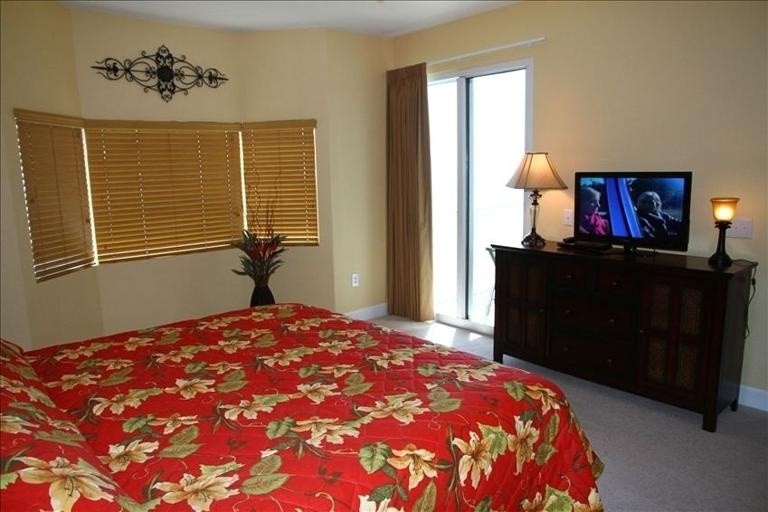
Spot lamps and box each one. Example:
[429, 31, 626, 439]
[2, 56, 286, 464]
[707, 198, 740, 269]
[506, 152, 568, 249]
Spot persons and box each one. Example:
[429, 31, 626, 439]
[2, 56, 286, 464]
[576, 185, 612, 236]
[634, 190, 682, 240]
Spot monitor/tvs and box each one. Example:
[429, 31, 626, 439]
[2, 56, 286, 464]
[573, 171, 692, 258]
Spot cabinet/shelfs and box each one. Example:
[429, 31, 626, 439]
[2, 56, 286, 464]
[489, 241, 759, 434]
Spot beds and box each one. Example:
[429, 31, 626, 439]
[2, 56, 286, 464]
[1, 302, 600, 512]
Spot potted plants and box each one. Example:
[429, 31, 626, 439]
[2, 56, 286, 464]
[230, 167, 292, 310]
